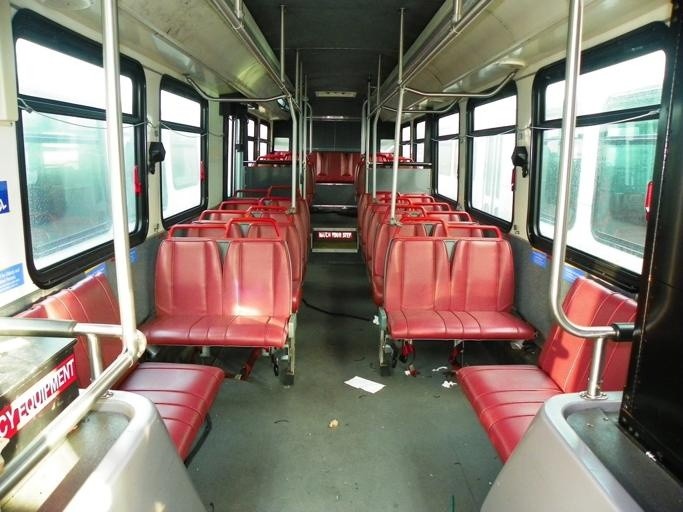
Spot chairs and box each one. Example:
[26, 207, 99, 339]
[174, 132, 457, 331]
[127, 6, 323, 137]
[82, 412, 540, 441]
[312, 148, 354, 186]
[354, 149, 636, 465]
[10, 149, 312, 463]
